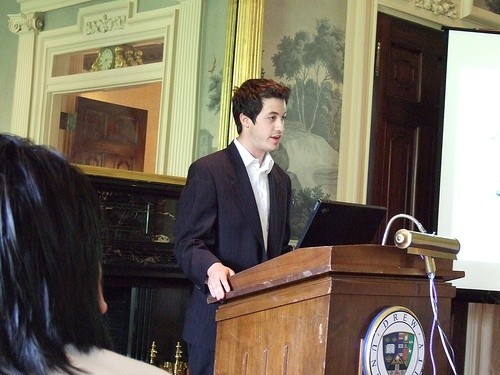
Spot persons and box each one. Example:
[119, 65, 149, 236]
[172, 79, 294, 375]
[0, 132, 175, 375]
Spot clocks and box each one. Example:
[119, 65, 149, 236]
[98, 48, 113, 70]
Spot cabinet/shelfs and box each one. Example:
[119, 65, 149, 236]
[67, 164, 195, 363]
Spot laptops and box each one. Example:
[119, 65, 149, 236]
[295, 199, 387, 250]
